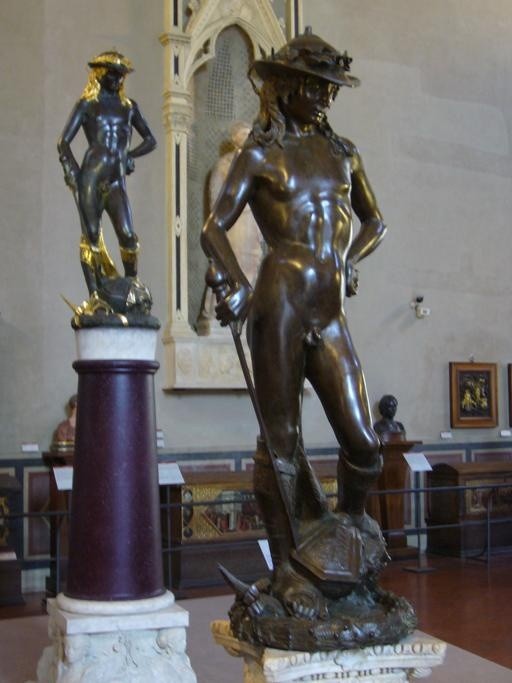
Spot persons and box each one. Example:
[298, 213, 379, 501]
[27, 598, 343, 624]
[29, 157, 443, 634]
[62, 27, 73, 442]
[56, 52, 157, 312]
[199, 122, 264, 322]
[53, 395, 77, 447]
[373, 395, 406, 442]
[198, 25, 387, 620]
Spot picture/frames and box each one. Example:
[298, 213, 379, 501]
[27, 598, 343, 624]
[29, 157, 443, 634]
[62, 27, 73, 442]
[450, 361, 500, 430]
